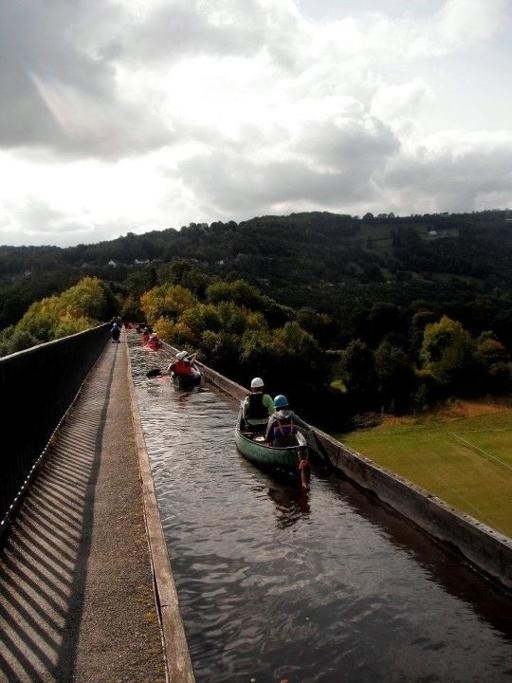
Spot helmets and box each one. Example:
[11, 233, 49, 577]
[251, 377, 265, 388]
[272, 394, 290, 409]
[175, 350, 188, 361]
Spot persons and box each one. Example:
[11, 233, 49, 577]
[165, 351, 200, 376]
[179, 350, 198, 370]
[110, 312, 160, 345]
[238, 375, 276, 433]
[264, 393, 314, 447]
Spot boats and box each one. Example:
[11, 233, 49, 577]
[170, 361, 201, 390]
[142, 333, 158, 351]
[235, 396, 308, 472]
